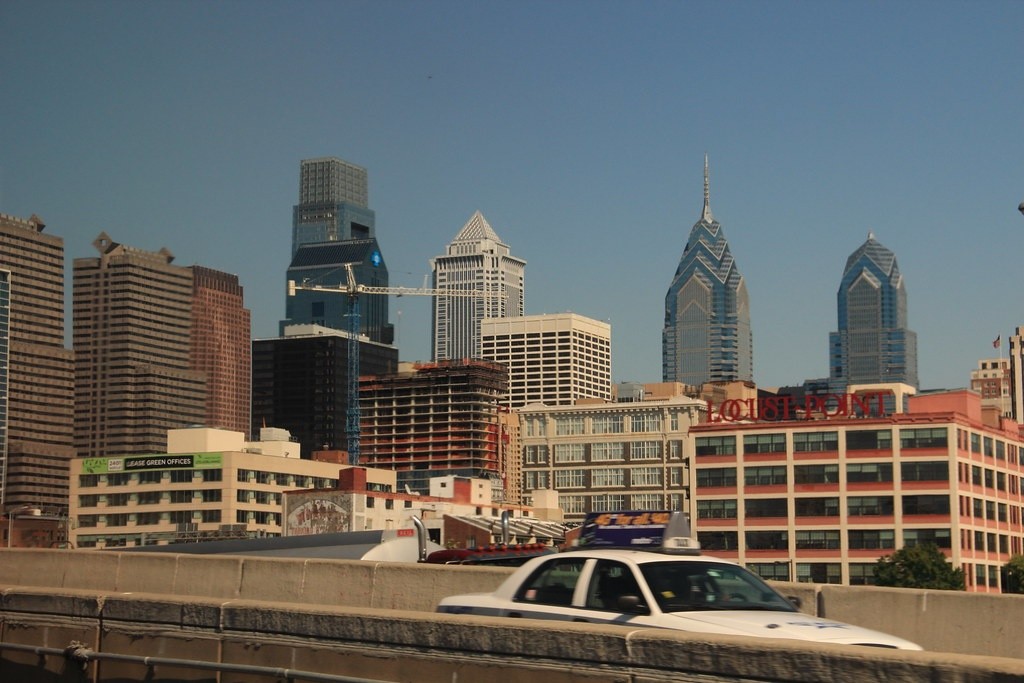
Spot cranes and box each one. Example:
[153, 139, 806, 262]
[286, 261, 508, 466]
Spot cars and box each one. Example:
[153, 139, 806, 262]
[435, 548, 925, 653]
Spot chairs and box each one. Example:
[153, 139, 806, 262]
[646, 571, 678, 605]
